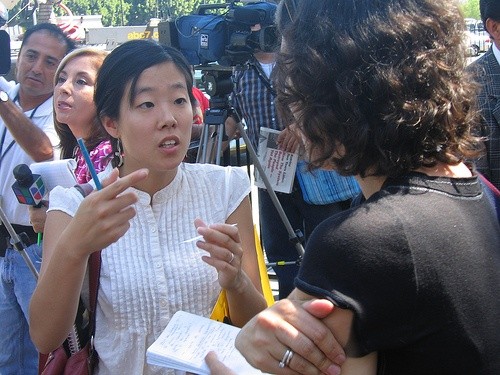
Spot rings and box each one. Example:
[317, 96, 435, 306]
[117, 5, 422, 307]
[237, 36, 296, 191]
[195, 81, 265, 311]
[279, 349, 290, 368]
[286, 350, 293, 365]
[229, 252, 234, 264]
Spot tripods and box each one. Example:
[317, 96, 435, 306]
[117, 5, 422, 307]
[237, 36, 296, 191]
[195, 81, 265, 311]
[197, 96, 306, 267]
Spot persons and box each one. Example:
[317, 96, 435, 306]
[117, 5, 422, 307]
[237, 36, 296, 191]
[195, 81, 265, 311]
[0, 0, 500, 375]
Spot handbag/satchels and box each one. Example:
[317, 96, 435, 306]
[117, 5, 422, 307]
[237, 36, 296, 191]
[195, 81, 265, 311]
[40, 306, 99, 375]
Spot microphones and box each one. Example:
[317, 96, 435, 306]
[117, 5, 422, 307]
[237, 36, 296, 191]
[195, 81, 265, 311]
[14, 163, 42, 210]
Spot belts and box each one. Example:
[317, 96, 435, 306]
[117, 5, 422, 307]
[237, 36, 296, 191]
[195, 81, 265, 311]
[0, 223, 38, 246]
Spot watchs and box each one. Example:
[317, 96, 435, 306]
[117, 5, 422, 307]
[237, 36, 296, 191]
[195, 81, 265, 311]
[0, 90, 8, 104]
